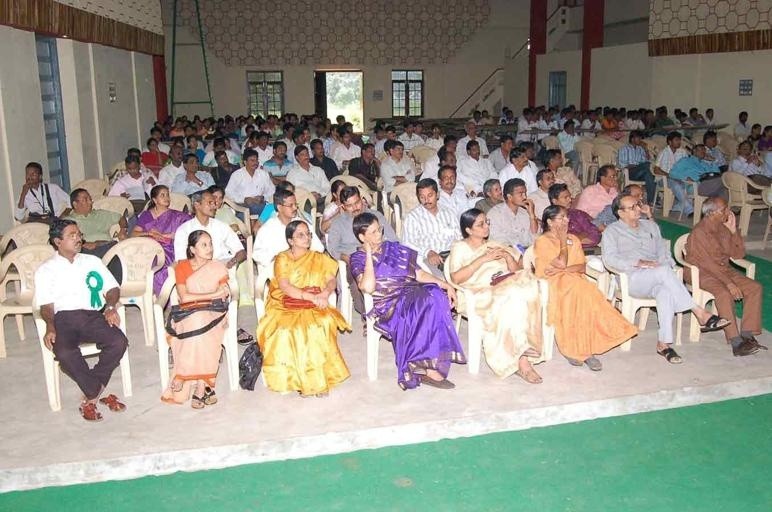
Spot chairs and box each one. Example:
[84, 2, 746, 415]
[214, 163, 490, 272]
[362, 259, 432, 382]
[579, 135, 771, 242]
[442, 251, 549, 374]
[153, 269, 240, 392]
[255, 259, 336, 384]
[31, 293, 134, 411]
[599, 251, 684, 351]
[675, 233, 756, 342]
[0, 175, 418, 357]
[522, 243, 610, 362]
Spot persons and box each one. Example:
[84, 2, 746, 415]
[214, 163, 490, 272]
[598, 184, 768, 365]
[349, 118, 490, 231]
[469, 105, 771, 220]
[13, 161, 128, 421]
[443, 165, 638, 384]
[249, 180, 352, 397]
[130, 184, 255, 409]
[321, 180, 467, 390]
[110, 112, 363, 214]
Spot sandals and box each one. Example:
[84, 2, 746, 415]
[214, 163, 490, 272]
[192, 384, 217, 408]
[585, 357, 602, 371]
[515, 356, 543, 384]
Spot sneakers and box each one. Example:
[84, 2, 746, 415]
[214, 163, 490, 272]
[700, 315, 767, 356]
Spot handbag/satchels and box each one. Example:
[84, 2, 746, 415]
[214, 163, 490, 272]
[239, 342, 264, 391]
[282, 286, 321, 309]
[167, 298, 229, 322]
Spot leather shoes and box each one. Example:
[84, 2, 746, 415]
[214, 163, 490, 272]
[100, 394, 126, 411]
[420, 373, 455, 389]
[78, 401, 103, 420]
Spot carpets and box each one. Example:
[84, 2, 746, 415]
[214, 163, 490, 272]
[655, 217, 772, 335]
[0, 391, 772, 512]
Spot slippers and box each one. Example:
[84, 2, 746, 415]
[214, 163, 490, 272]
[656, 347, 683, 366]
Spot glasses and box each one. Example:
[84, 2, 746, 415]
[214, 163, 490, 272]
[474, 219, 491, 227]
[616, 201, 641, 215]
